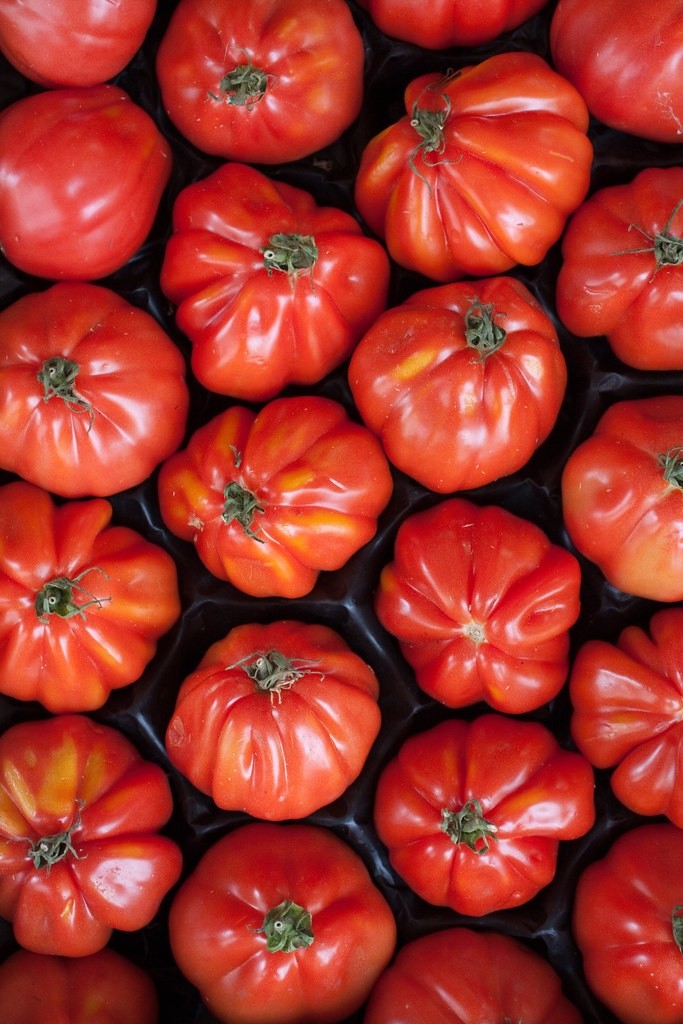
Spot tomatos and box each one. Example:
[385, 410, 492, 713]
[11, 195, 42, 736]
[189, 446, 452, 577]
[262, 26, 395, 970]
[0, 0, 683, 1024]
